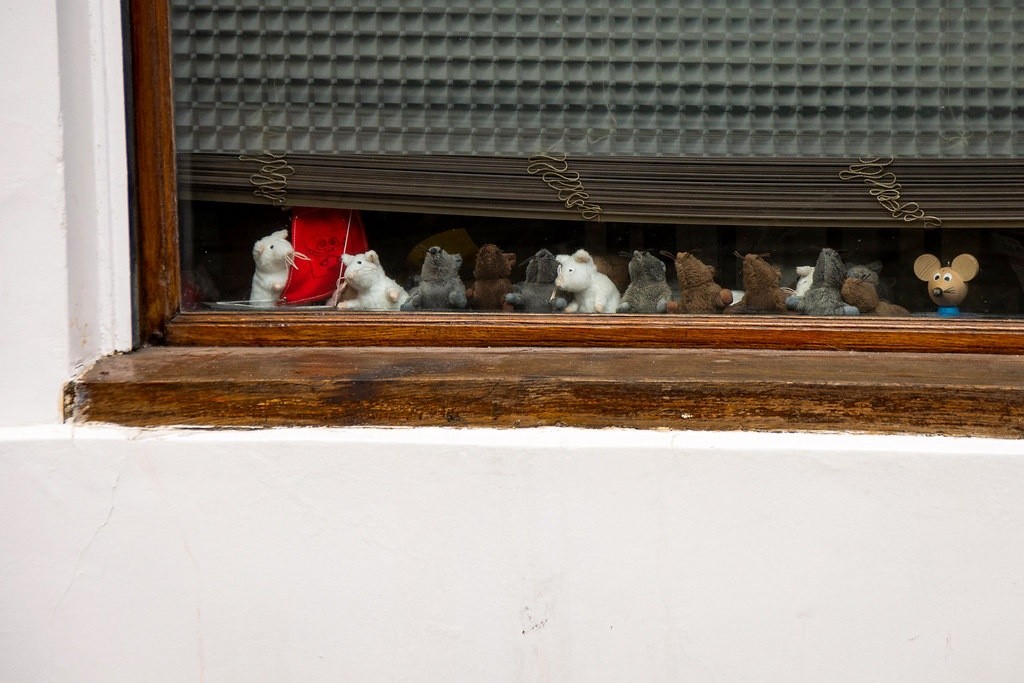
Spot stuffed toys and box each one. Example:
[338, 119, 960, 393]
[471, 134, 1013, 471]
[249, 228, 310, 307]
[337, 244, 914, 318]
